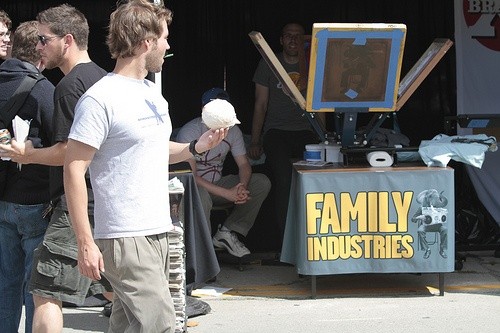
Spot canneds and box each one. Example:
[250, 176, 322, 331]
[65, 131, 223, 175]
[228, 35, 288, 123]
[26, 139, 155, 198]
[0, 129, 13, 157]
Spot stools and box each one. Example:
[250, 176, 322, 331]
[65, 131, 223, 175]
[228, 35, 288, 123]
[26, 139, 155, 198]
[211, 205, 245, 270]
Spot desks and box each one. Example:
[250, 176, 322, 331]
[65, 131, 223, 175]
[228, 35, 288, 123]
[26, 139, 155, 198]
[280, 163, 455, 299]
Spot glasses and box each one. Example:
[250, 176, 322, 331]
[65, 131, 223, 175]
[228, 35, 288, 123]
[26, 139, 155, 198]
[37, 35, 75, 46]
[0, 31, 11, 39]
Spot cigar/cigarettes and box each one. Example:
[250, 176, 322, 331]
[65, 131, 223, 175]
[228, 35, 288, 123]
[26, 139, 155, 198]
[2, 45, 11, 48]
[163, 52, 174, 60]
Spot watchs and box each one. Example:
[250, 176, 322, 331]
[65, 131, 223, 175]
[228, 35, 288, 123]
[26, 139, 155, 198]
[189, 140, 205, 158]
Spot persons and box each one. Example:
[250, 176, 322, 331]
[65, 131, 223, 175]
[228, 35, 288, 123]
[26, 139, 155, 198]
[411, 188, 448, 259]
[0, 20, 55, 333]
[64, 0, 241, 333]
[0, 3, 114, 333]
[0, 11, 12, 68]
[173, 88, 272, 283]
[247, 20, 326, 246]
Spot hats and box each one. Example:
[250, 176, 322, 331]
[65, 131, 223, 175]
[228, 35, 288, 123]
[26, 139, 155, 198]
[201, 88, 231, 106]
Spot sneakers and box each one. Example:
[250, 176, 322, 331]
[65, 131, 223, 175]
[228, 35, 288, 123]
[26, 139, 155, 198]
[212, 224, 251, 259]
